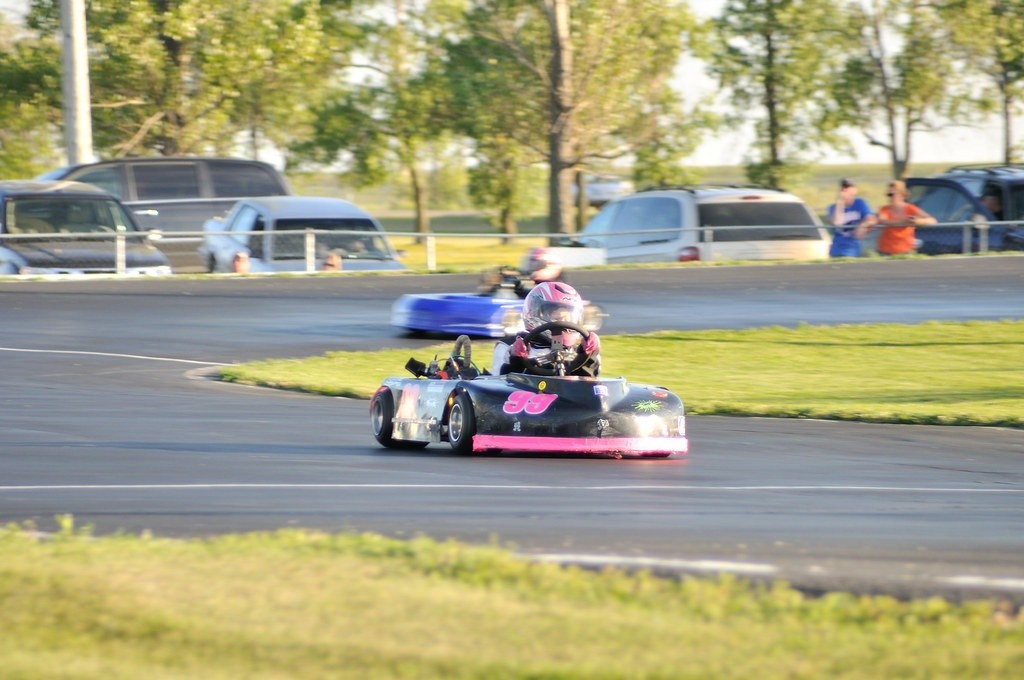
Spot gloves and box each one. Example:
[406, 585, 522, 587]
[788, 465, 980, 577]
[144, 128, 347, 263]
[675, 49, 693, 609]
[581, 332, 600, 355]
[514, 337, 532, 359]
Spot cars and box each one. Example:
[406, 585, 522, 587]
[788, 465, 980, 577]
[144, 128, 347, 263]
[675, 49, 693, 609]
[195, 196, 406, 276]
[900, 168, 1023, 256]
[31, 156, 295, 274]
[0, 180, 174, 279]
[570, 174, 635, 208]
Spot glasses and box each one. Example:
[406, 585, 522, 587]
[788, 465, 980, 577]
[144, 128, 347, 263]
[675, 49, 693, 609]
[885, 192, 896, 198]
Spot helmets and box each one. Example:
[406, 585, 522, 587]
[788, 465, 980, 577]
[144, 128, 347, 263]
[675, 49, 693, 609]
[522, 281, 585, 347]
[527, 246, 563, 281]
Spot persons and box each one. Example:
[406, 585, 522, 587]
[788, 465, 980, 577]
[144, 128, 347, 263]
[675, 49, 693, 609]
[320, 254, 341, 271]
[871, 181, 939, 255]
[827, 180, 880, 256]
[950, 192, 1003, 227]
[486, 243, 568, 298]
[233, 251, 251, 272]
[491, 281, 601, 378]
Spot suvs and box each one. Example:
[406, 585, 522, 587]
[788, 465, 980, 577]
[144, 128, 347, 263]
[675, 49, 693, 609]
[552, 184, 832, 268]
